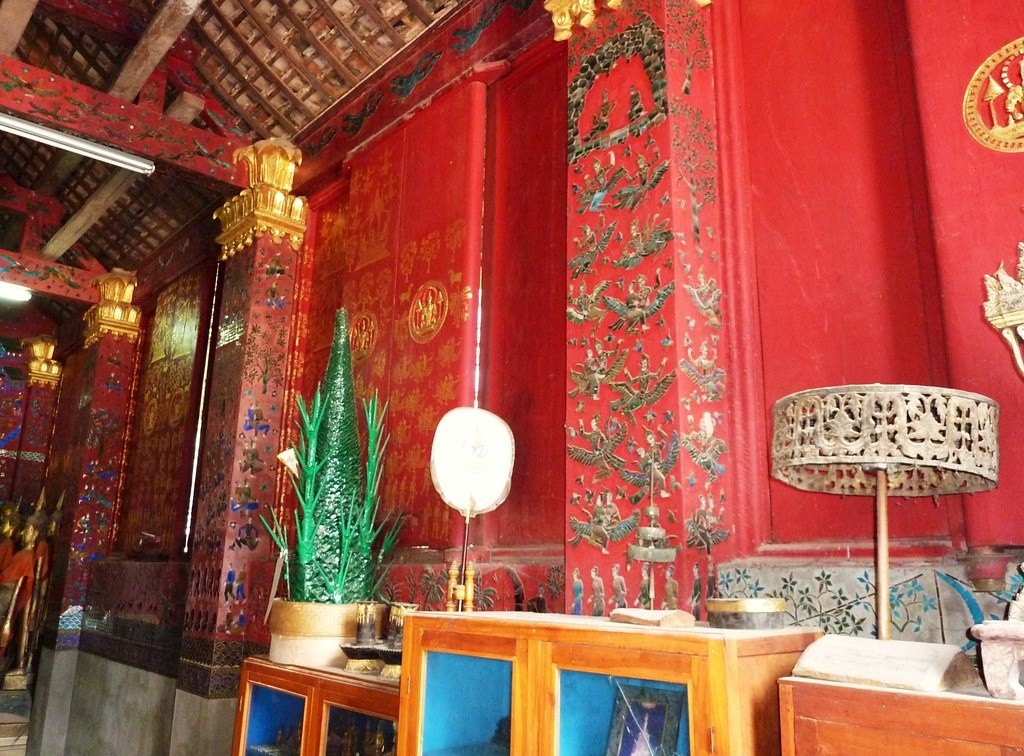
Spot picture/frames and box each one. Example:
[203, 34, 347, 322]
[604, 684, 685, 756]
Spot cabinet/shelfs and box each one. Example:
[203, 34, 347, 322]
[233, 604, 1024, 756]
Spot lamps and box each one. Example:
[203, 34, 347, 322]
[0, 112, 156, 176]
[768, 384, 999, 642]
